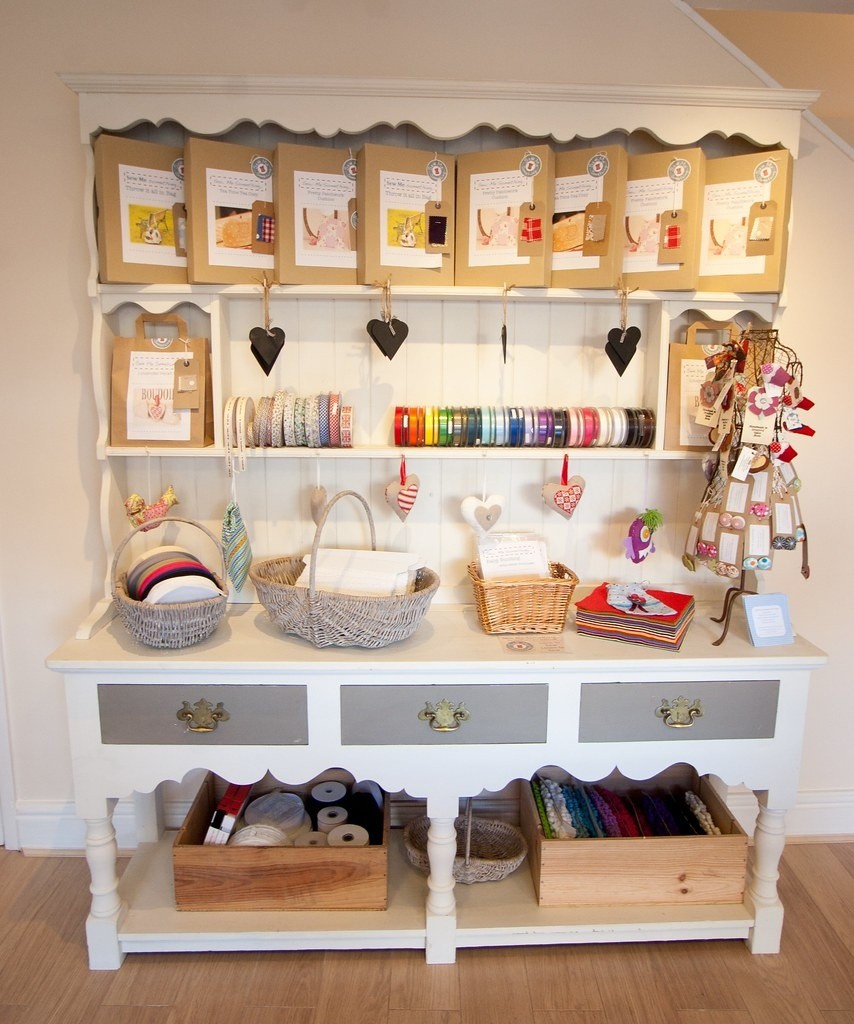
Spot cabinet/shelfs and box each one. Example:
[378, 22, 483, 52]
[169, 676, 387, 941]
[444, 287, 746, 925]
[47, 70, 830, 972]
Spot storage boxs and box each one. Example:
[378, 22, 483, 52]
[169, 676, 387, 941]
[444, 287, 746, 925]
[522, 765, 749, 907]
[93, 133, 793, 295]
[172, 771, 390, 912]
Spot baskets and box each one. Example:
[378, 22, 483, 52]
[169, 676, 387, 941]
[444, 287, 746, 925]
[468, 556, 579, 635]
[111, 515, 230, 648]
[247, 490, 439, 648]
[404, 796, 525, 884]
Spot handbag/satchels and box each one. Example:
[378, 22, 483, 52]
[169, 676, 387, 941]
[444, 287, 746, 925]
[106, 313, 212, 450]
[221, 500, 253, 594]
